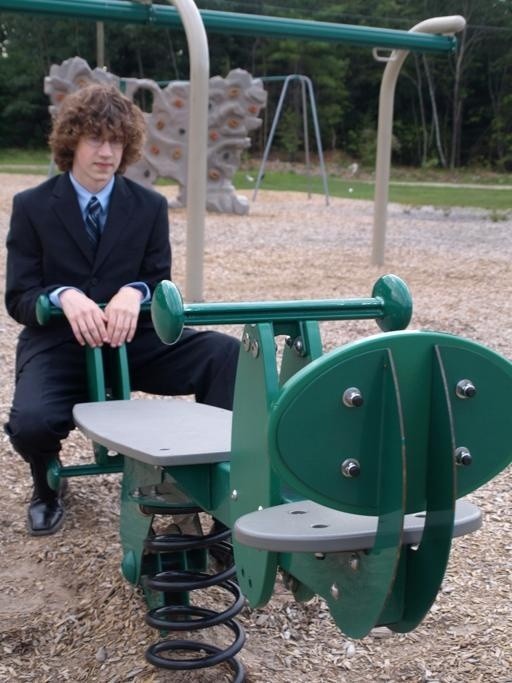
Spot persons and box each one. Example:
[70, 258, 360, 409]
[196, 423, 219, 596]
[5, 84, 241, 565]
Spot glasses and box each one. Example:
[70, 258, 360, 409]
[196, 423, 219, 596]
[79, 133, 129, 149]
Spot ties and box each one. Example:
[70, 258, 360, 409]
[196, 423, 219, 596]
[84, 196, 104, 253]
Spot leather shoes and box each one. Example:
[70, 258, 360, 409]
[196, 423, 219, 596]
[207, 517, 236, 567]
[24, 464, 69, 538]
